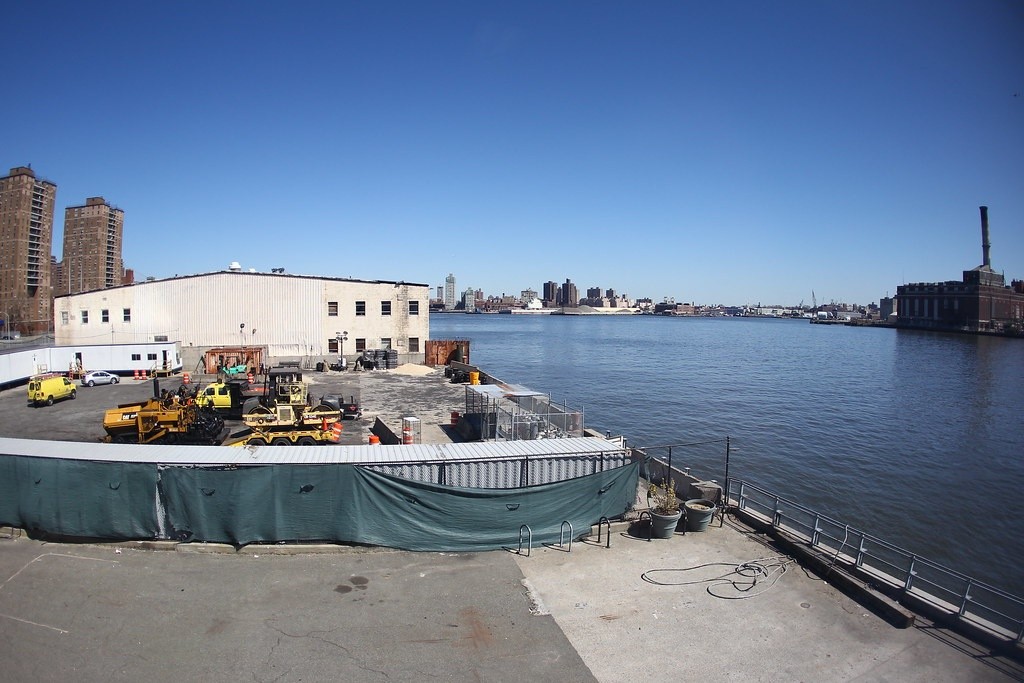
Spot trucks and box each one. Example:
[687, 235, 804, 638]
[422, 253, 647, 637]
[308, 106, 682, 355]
[192, 378, 270, 419]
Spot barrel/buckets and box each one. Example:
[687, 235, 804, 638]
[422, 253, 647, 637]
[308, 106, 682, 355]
[362, 349, 397, 370]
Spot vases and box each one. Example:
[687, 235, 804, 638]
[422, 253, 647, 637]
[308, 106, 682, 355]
[685, 499, 716, 532]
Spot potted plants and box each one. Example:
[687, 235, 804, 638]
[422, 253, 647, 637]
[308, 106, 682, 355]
[647, 478, 682, 539]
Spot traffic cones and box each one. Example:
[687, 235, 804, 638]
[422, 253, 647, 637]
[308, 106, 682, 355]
[322, 417, 328, 431]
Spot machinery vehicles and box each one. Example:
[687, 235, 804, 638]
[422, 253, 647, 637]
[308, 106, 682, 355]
[95, 377, 231, 447]
[241, 361, 363, 433]
[227, 380, 344, 446]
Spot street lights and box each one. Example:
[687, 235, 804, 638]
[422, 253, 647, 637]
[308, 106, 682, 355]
[37, 310, 50, 335]
[251, 328, 257, 364]
[239, 322, 246, 366]
[0, 311, 11, 341]
[335, 330, 349, 366]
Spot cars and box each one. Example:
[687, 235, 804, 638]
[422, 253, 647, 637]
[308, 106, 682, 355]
[220, 363, 247, 375]
[81, 370, 121, 387]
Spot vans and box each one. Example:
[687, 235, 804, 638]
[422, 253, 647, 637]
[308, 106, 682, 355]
[27, 372, 77, 407]
